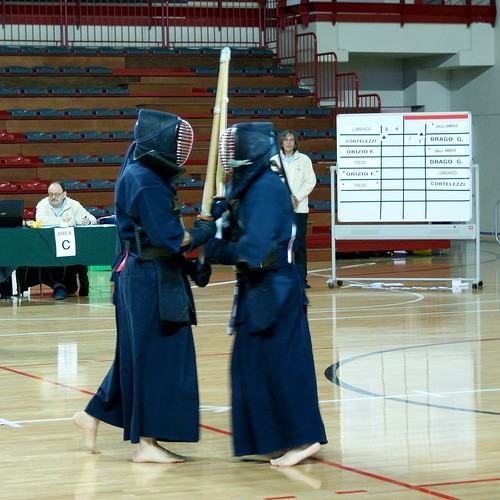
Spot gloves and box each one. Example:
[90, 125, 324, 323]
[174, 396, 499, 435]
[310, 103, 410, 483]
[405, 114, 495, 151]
[181, 258, 212, 288]
[184, 216, 217, 254]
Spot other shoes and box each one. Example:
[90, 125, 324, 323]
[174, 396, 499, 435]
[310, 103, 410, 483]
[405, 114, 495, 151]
[53, 286, 67, 300]
[304, 283, 312, 288]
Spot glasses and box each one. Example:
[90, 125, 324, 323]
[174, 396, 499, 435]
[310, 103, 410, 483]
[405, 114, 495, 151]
[48, 192, 64, 197]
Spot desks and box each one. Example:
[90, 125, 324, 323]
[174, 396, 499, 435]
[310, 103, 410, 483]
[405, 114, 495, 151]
[0, 225, 116, 295]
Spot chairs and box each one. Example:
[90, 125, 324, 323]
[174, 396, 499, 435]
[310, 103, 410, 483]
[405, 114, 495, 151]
[0, 47, 339, 233]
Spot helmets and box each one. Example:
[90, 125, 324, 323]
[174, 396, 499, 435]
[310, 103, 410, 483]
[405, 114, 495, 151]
[217, 120, 278, 180]
[134, 109, 194, 167]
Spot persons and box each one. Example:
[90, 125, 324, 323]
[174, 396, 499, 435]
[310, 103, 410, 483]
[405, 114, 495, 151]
[204, 121, 329, 467]
[72, 108, 216, 462]
[35, 182, 97, 299]
[270, 130, 317, 288]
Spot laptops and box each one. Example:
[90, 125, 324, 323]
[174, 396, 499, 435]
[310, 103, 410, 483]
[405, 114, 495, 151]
[0, 199, 24, 227]
